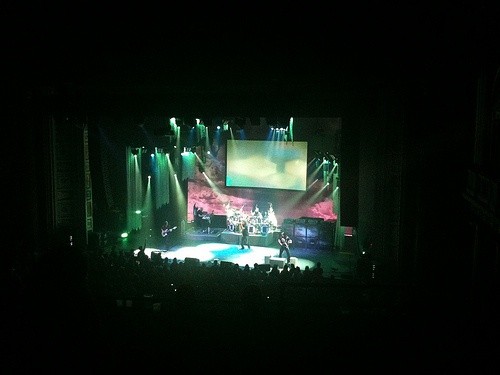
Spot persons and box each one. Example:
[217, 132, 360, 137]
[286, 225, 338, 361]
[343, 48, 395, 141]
[240, 220, 250, 250]
[191, 200, 277, 234]
[68, 238, 323, 311]
[160, 220, 170, 250]
[278, 231, 292, 264]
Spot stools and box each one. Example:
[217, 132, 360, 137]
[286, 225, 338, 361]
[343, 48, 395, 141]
[269, 257, 287, 268]
[289, 256, 298, 268]
[265, 256, 271, 264]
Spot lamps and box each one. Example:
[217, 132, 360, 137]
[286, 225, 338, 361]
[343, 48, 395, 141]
[132, 116, 341, 192]
